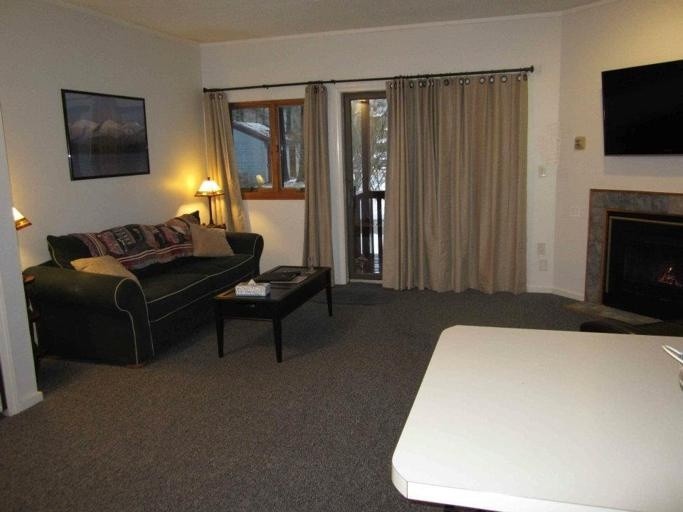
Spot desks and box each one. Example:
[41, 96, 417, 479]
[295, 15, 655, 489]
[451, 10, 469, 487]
[391, 325, 682, 512]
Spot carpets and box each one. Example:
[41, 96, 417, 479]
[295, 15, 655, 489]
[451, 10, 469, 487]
[310, 285, 394, 306]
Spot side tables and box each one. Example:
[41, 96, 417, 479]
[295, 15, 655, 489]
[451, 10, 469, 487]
[22, 274, 39, 360]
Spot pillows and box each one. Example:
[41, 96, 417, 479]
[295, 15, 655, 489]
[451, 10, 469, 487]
[46, 211, 236, 287]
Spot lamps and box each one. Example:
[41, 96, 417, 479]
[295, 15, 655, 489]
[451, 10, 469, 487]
[195, 175, 225, 227]
[12, 207, 32, 280]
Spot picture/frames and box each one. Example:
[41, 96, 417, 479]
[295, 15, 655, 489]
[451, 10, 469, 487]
[60, 89, 150, 181]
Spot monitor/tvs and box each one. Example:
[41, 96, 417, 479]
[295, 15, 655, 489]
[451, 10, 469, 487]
[601, 59, 683, 155]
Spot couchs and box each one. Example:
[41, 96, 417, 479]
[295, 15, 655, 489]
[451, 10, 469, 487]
[24, 210, 264, 369]
